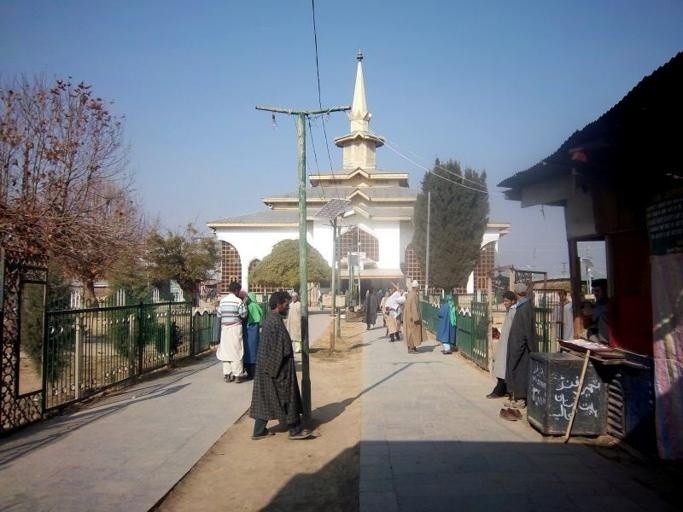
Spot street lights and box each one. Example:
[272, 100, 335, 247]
[314, 198, 356, 349]
[256, 104, 311, 428]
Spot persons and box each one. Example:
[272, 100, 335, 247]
[579, 279, 608, 344]
[318, 293, 324, 311]
[214, 281, 249, 384]
[286, 291, 302, 353]
[248, 291, 314, 441]
[484, 291, 519, 399]
[361, 280, 429, 354]
[338, 287, 348, 295]
[436, 294, 457, 354]
[551, 290, 573, 340]
[503, 282, 539, 409]
[237, 290, 263, 380]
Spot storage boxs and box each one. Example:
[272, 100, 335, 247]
[526, 352, 605, 436]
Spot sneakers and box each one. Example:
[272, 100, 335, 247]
[224, 374, 248, 383]
[390, 332, 401, 343]
[441, 350, 452, 354]
[486, 393, 499, 400]
[500, 396, 526, 422]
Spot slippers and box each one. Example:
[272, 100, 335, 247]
[252, 430, 273, 440]
[287, 430, 311, 440]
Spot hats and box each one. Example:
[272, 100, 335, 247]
[410, 279, 419, 288]
[514, 282, 528, 295]
